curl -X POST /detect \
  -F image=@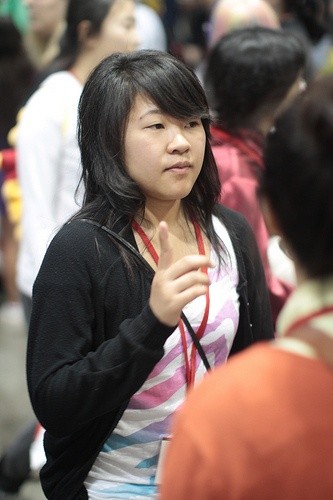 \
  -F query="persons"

[154,69,333,500]
[201,25,309,335]
[18,0,72,95]
[0,0,144,500]
[24,48,277,500]
[132,0,333,136]
[0,15,42,332]
